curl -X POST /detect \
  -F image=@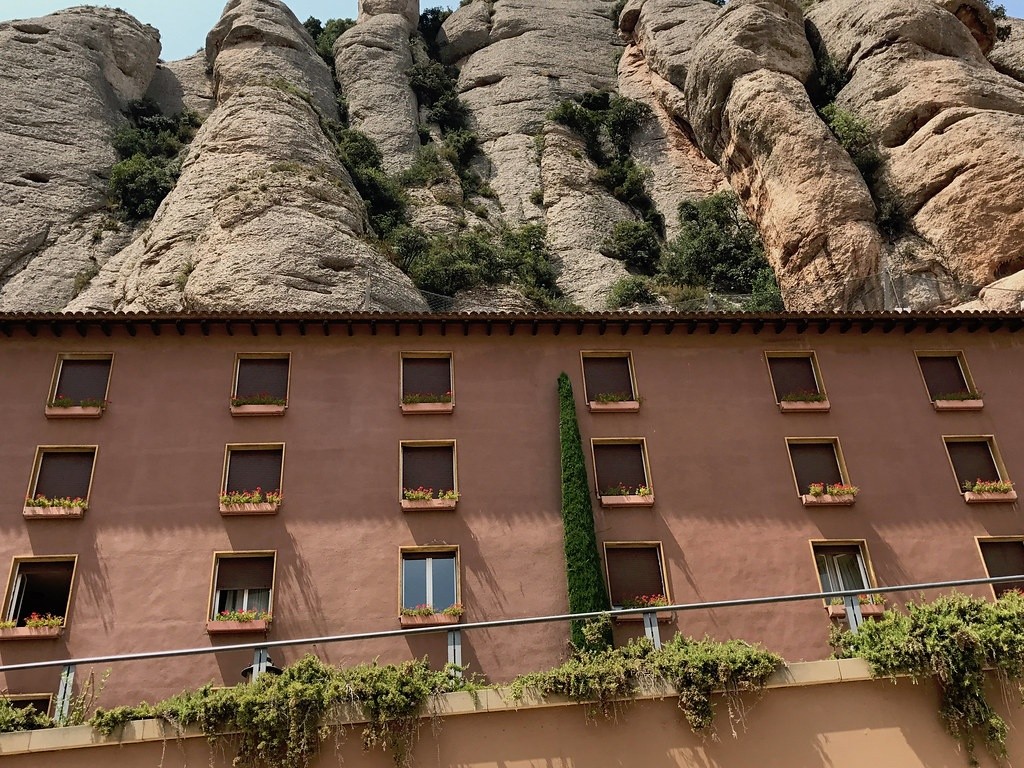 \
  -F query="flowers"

[601,481,650,495]
[857,594,886,604]
[934,386,986,402]
[25,495,90,510]
[399,391,453,404]
[23,612,64,627]
[215,609,271,623]
[45,394,112,410]
[228,391,286,407]
[782,389,827,404]
[218,485,280,507]
[596,390,644,405]
[397,604,467,617]
[962,477,1016,494]
[808,482,859,498]
[402,486,461,502]
[621,595,668,606]
